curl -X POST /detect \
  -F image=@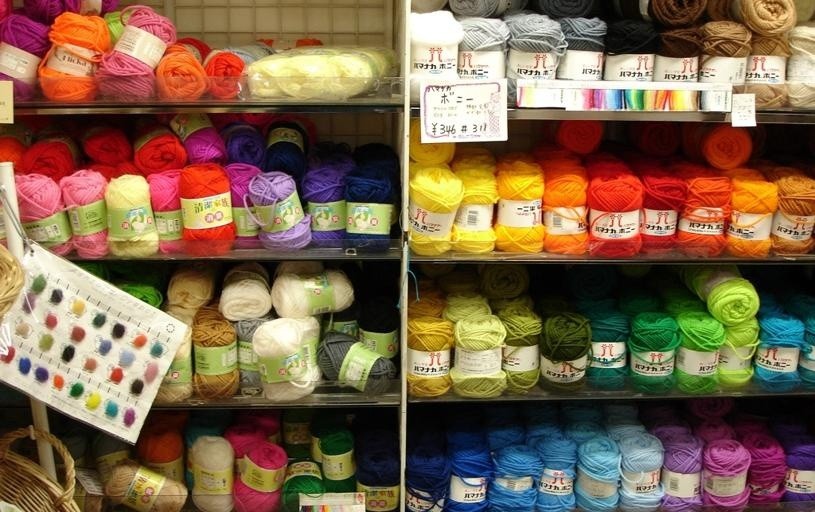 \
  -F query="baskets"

[0,427,82,511]
[0,246,25,325]
